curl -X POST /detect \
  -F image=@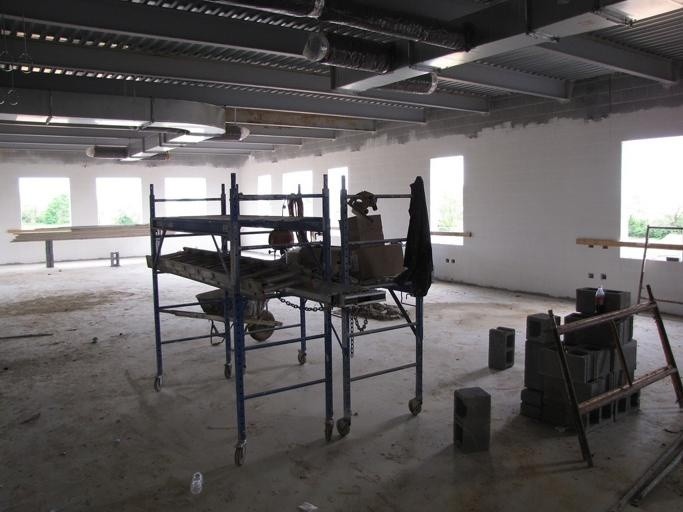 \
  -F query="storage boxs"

[285,214,404,281]
